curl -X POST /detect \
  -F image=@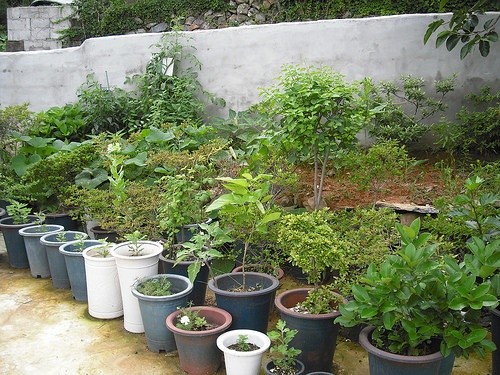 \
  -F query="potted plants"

[0,128,500,375]
